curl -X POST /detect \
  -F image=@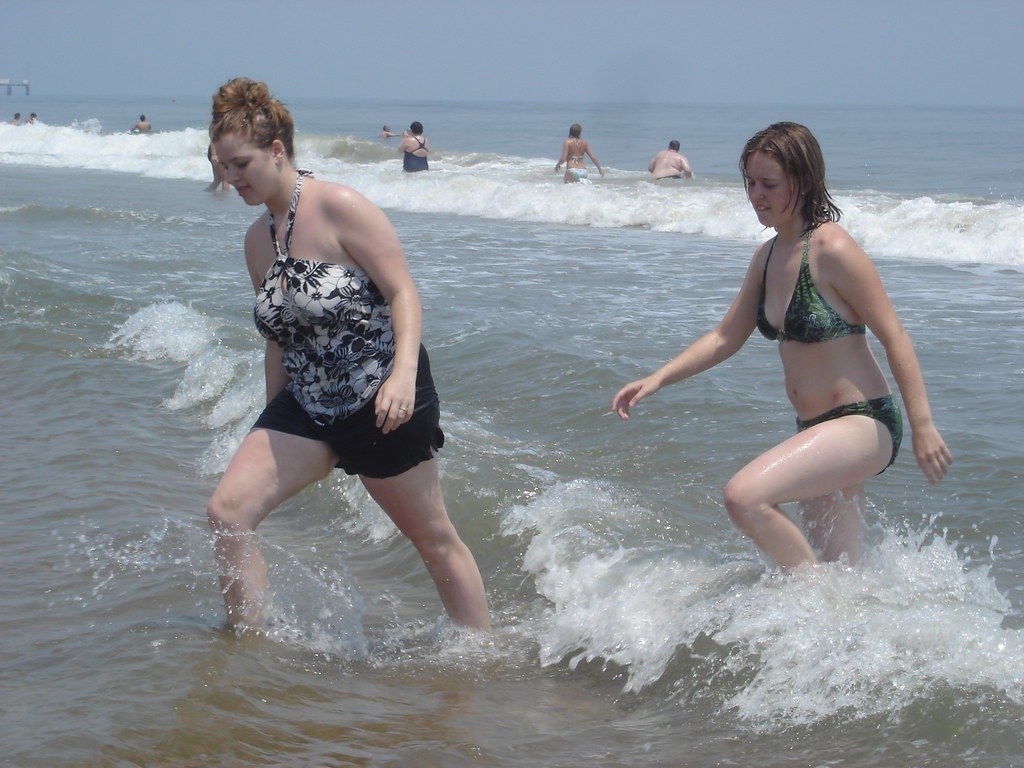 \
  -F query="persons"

[206,78,490,628]
[649,139,690,178]
[380,125,397,137]
[208,138,229,190]
[398,121,429,171]
[613,121,953,571]
[11,113,20,125]
[556,123,604,182]
[26,113,37,123]
[131,115,151,132]
